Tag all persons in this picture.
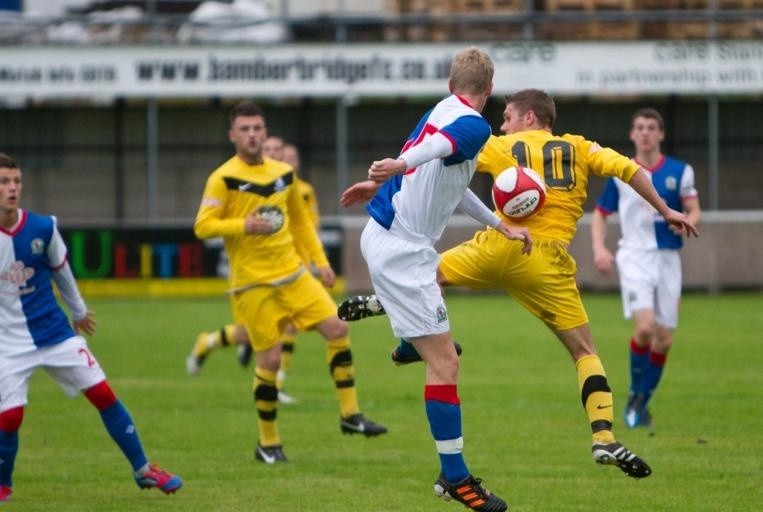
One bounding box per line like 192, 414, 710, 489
237, 145, 319, 366
195, 102, 388, 461
1, 152, 181, 499
187, 133, 333, 414
358, 47, 537, 510
590, 108, 702, 432
336, 87, 698, 480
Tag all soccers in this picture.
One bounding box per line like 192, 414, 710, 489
492, 165, 544, 220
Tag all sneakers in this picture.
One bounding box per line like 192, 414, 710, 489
134, 464, 183, 496
1, 486, 13, 501
624, 398, 643, 428
434, 473, 507, 512
340, 412, 388, 438
628, 395, 652, 426
254, 439, 287, 464
390, 342, 462, 366
592, 441, 652, 478
337, 294, 385, 322
186, 331, 297, 405
